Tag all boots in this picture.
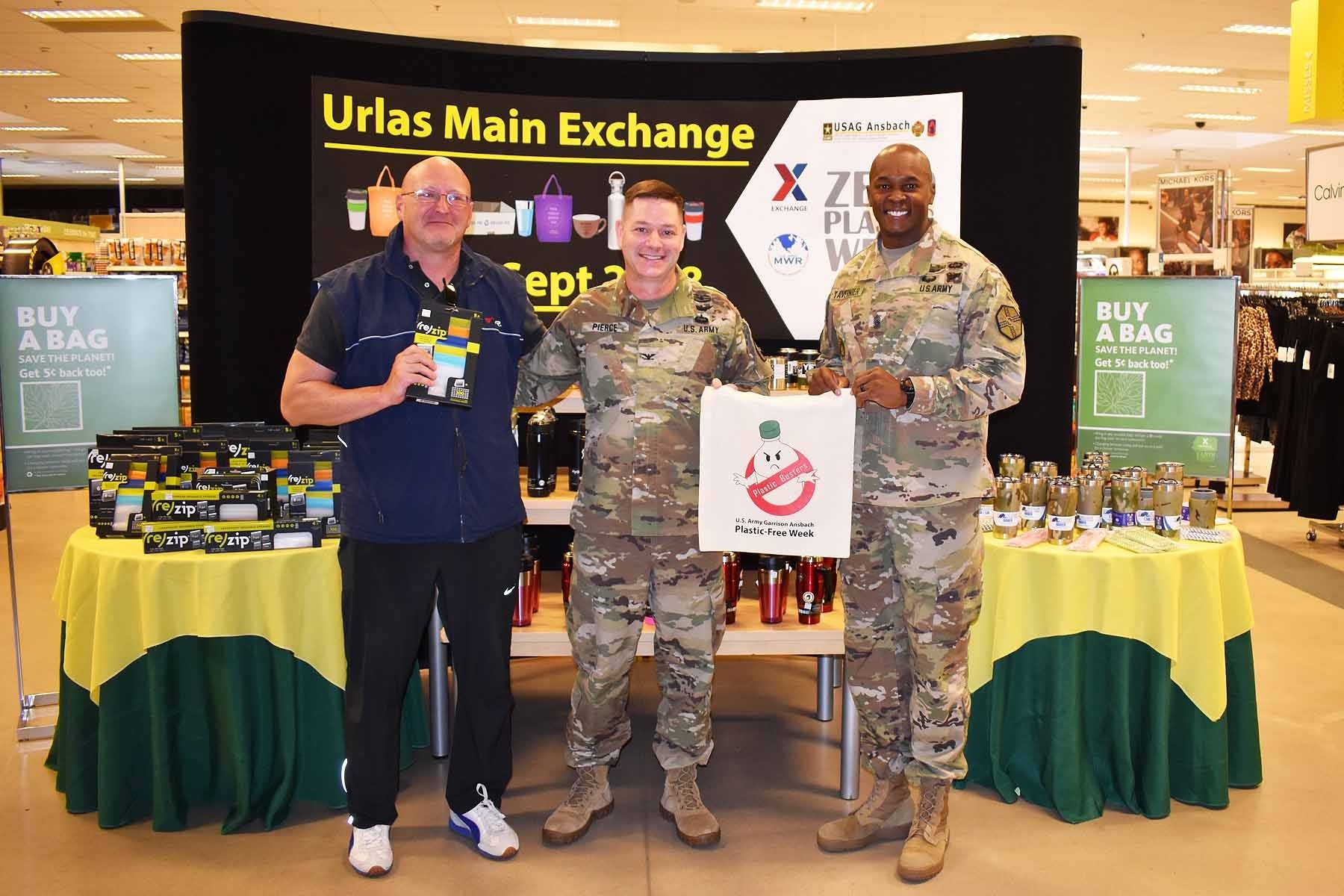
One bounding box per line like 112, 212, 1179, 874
899, 776, 951, 881
543, 765, 617, 844
659, 764, 721, 846
817, 768, 915, 852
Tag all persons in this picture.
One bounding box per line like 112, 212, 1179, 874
1127, 249, 1148, 276
1078, 217, 1090, 240
514, 180, 774, 848
809, 143, 1026, 884
1180, 188, 1213, 247
1089, 219, 1117, 239
280, 156, 547, 878
1265, 250, 1292, 269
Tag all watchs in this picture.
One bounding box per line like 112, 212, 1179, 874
900, 377, 915, 408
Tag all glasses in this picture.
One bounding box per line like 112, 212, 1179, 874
442, 277, 459, 306
401, 189, 475, 209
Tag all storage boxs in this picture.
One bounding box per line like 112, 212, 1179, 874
406, 300, 483, 410
87, 423, 341, 554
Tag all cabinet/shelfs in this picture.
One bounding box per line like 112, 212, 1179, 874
107, 262, 191, 404
428, 466, 862, 801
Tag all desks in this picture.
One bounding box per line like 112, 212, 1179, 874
951, 516, 1263, 822
43, 523, 428, 833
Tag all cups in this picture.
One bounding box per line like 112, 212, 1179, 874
573, 214, 606, 239
980, 451, 1217, 547
516, 200, 534, 237
510, 531, 575, 627
764, 355, 787, 391
347, 189, 367, 231
684, 201, 705, 241
722, 552, 836, 624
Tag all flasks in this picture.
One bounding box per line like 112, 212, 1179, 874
607, 171, 627, 250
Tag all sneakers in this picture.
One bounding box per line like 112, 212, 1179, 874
449, 785, 520, 862
348, 825, 393, 876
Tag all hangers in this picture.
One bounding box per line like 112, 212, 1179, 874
1239, 283, 1344, 327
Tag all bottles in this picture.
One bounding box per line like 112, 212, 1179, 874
510, 407, 587, 497
779, 347, 820, 392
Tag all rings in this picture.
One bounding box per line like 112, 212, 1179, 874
860, 385, 865, 392
